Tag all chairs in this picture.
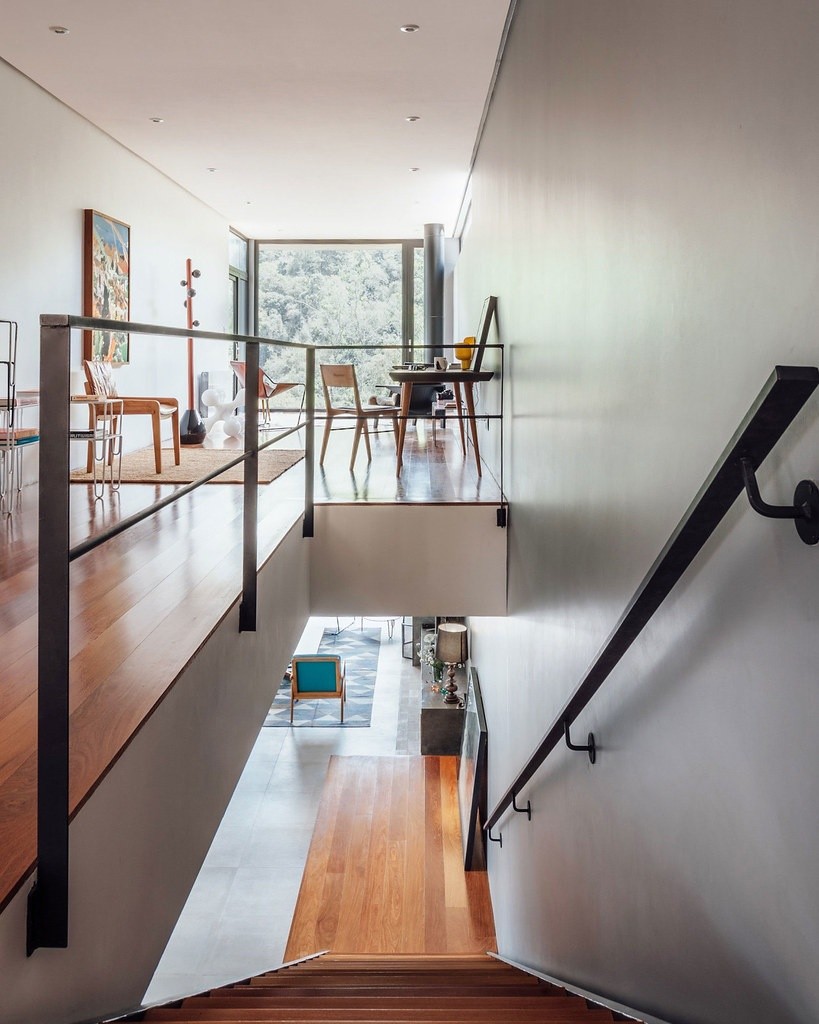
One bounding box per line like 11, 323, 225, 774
228, 358, 306, 433
319, 361, 401, 468
290, 653, 347, 725
82, 358, 181, 475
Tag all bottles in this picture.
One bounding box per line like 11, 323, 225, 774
433, 656, 444, 683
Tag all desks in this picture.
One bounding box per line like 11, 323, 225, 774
360, 617, 400, 639
388, 368, 495, 479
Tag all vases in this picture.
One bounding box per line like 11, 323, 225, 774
431, 665, 444, 682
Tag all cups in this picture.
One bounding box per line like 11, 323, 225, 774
434, 357, 446, 372
431, 682, 439, 694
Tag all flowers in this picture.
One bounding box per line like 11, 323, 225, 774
416, 634, 445, 671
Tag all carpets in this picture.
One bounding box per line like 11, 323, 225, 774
70, 447, 306, 484
262, 627, 382, 728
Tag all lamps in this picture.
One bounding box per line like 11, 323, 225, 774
434, 623, 469, 705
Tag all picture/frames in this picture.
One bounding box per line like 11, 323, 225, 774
85, 208, 132, 365
456, 666, 488, 871
468, 295, 497, 372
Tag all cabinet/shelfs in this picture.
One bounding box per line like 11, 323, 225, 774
0, 320, 124, 517
420, 622, 468, 756
